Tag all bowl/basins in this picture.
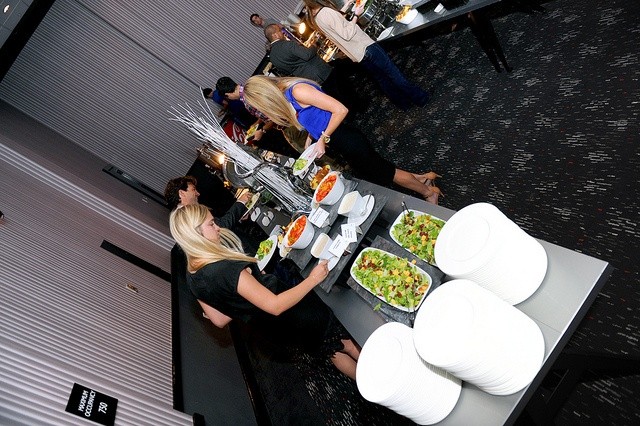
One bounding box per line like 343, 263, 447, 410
313, 170, 345, 205
284, 214, 315, 250
310, 233, 334, 261
396, 8, 418, 24
337, 191, 365, 218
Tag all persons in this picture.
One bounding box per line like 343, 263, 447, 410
243, 74, 445, 206
304, 0, 435, 110
203, 87, 259, 127
250, 14, 285, 30
264, 24, 367, 111
216, 77, 301, 159
169, 205, 360, 383
164, 176, 270, 258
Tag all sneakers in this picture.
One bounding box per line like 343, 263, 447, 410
420, 87, 434, 110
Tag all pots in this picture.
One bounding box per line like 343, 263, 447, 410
356, 0, 379, 26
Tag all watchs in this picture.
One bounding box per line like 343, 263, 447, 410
320, 131, 330, 144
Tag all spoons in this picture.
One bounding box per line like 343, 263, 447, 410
360, 192, 372, 216
262, 211, 274, 225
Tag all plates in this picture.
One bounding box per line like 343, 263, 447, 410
279, 233, 292, 257
316, 257, 340, 272
374, 26, 393, 41
244, 124, 259, 144
414, 280, 545, 397
250, 207, 261, 222
262, 207, 281, 226
260, 150, 267, 158
308, 166, 322, 181
434, 203, 547, 306
356, 322, 463, 426
292, 143, 319, 175
347, 195, 375, 227
434, 3, 444, 12
350, 247, 433, 313
323, 46, 338, 62
269, 225, 282, 239
284, 158, 295, 168
277, 231, 287, 248
298, 168, 309, 179
352, 0, 367, 12
237, 189, 249, 200
243, 192, 260, 217
254, 235, 277, 272
389, 208, 448, 268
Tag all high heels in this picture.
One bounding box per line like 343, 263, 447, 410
425, 186, 445, 205
423, 172, 442, 187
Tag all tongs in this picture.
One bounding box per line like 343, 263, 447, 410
401, 201, 416, 228
403, 281, 417, 327
